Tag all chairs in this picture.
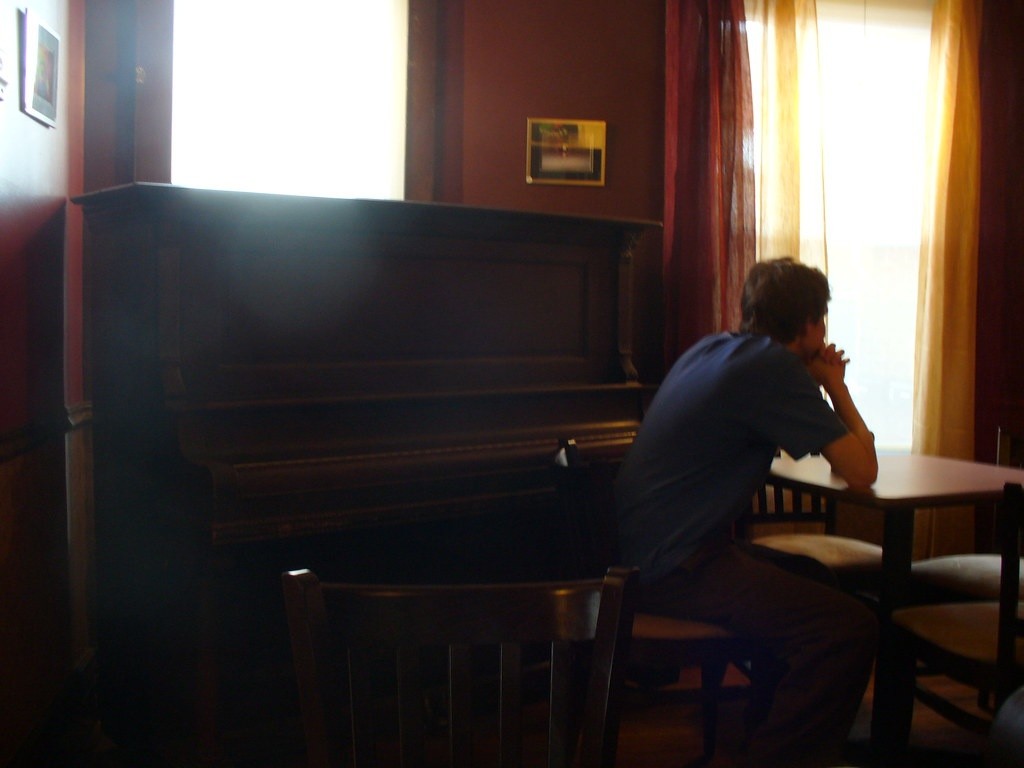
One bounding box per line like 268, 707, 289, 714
747, 447, 881, 689
548, 440, 742, 768
283, 565, 641, 768
881, 482, 1024, 768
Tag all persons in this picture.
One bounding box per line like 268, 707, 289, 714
613, 258, 880, 767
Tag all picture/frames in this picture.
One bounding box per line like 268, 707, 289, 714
524, 116, 609, 186
23, 7, 62, 130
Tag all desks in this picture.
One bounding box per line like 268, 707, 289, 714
764, 452, 1024, 768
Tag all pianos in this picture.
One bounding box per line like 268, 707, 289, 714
68, 182, 660, 768
